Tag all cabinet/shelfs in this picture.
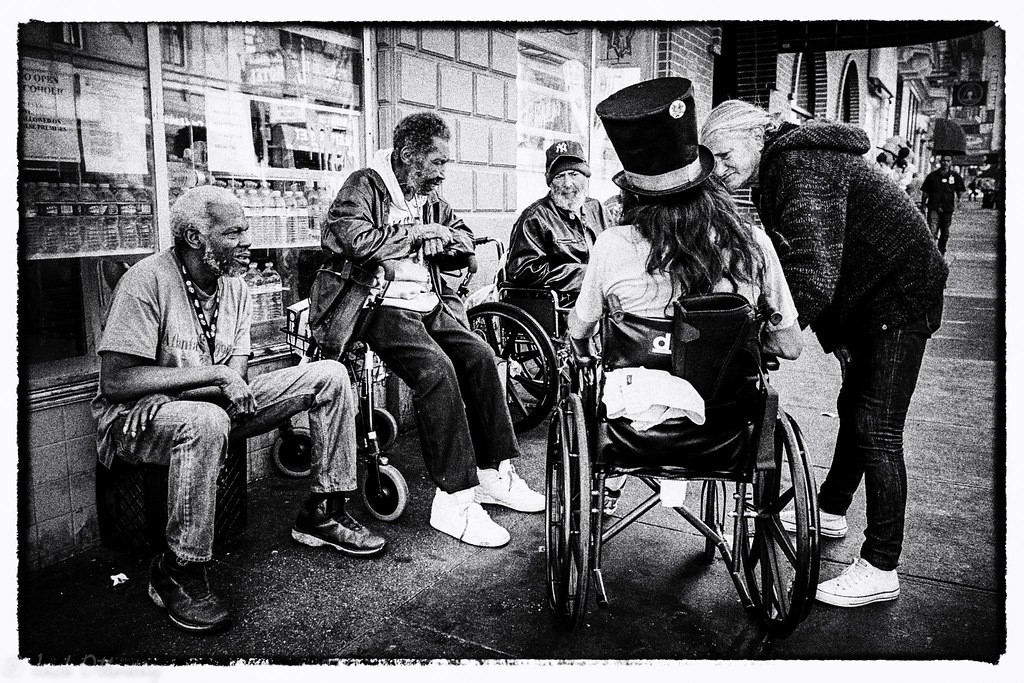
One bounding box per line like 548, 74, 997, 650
17, 21, 373, 259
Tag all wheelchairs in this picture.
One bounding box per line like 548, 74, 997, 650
270, 237, 572, 525
543, 289, 821, 635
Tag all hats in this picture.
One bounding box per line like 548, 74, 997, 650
897, 159, 908, 169
877, 153, 890, 164
595, 77, 717, 199
898, 147, 910, 158
545, 140, 592, 185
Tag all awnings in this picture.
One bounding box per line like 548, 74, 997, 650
934, 117, 966, 155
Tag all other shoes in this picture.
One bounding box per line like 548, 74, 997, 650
591, 472, 628, 516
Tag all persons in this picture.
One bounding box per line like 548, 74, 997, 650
702, 98, 949, 608
507, 141, 616, 312
875, 146, 979, 258
308, 112, 547, 548
564, 76, 805, 360
89, 185, 389, 633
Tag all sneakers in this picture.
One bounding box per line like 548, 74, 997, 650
815, 557, 900, 607
291, 492, 387, 554
148, 552, 229, 629
779, 507, 848, 538
474, 458, 545, 512
429, 487, 511, 547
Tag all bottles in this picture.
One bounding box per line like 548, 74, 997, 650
240, 262, 283, 322
20, 181, 155, 255
168, 137, 331, 243
